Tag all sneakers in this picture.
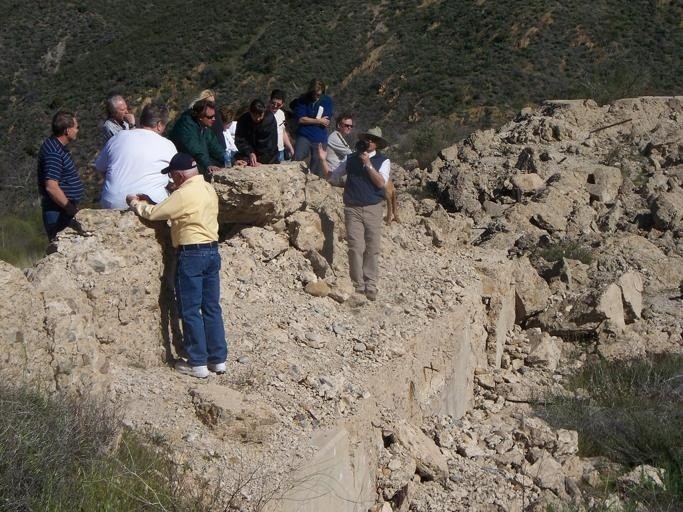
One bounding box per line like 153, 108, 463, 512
174, 359, 209, 379
367, 292, 378, 302
208, 361, 227, 375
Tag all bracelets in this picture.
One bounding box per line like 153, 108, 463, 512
367, 165, 373, 170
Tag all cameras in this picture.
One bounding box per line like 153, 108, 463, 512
356, 140, 370, 151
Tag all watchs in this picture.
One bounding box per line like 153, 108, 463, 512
129, 123, 136, 128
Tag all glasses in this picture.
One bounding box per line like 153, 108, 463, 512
202, 114, 218, 118
271, 100, 282, 107
340, 123, 355, 127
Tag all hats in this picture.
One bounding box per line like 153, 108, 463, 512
160, 152, 198, 174
358, 124, 390, 150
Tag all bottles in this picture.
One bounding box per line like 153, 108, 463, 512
223, 150, 231, 169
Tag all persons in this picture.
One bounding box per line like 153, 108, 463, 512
96, 103, 178, 209
103, 94, 137, 144
37, 110, 84, 242
164, 89, 297, 166
127, 152, 228, 378
328, 124, 390, 301
325, 113, 355, 187
292, 78, 333, 175
